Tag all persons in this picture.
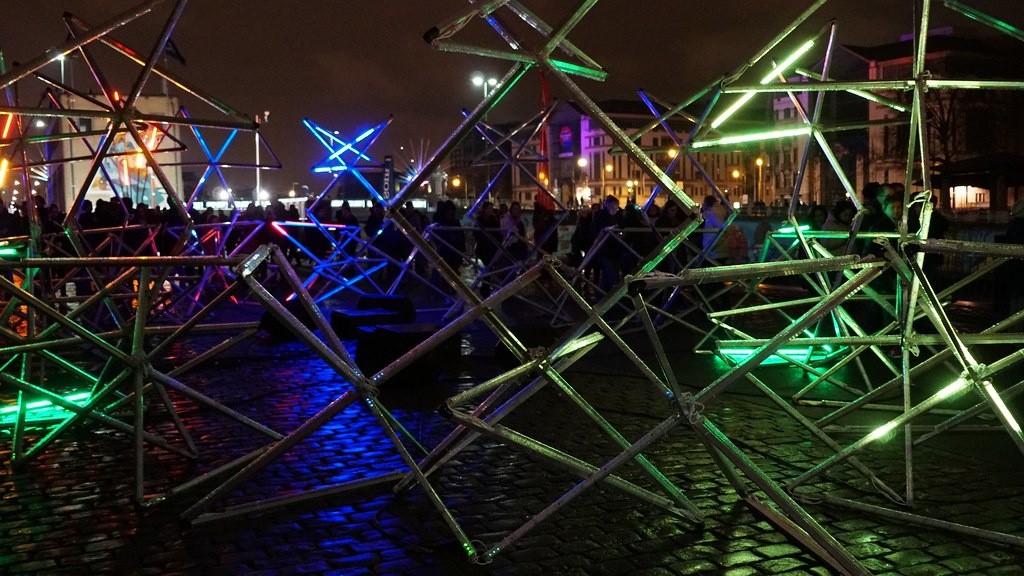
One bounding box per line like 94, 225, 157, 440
1, 180, 959, 333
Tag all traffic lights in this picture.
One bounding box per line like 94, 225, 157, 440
452, 177, 468, 200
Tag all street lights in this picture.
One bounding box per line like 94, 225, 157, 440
601, 163, 614, 202
469, 73, 498, 203
756, 158, 763, 202
